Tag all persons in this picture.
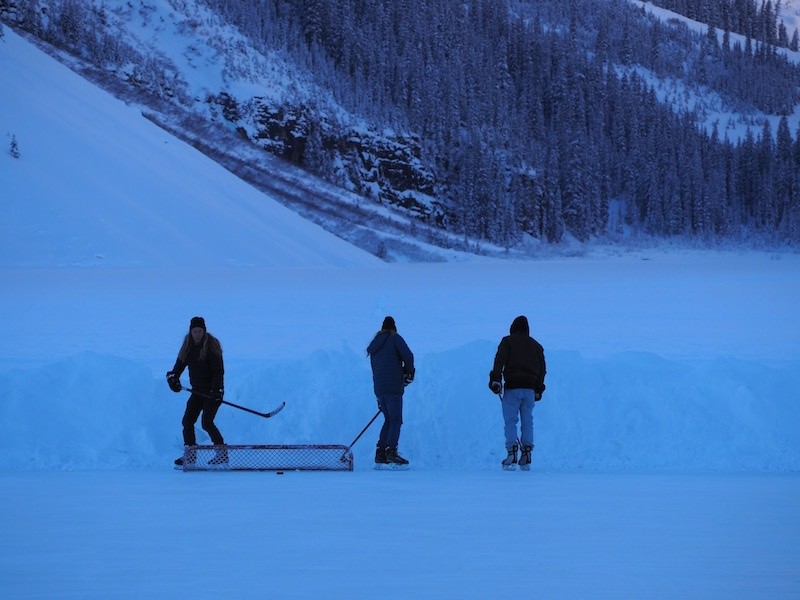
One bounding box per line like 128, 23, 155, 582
488, 316, 546, 471
166, 317, 228, 469
366, 316, 416, 471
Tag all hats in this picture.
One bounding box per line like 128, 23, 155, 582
190, 316, 206, 331
382, 316, 397, 332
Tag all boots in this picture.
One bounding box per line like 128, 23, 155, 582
518, 445, 531, 470
208, 443, 229, 464
376, 445, 387, 463
175, 443, 198, 465
385, 446, 410, 463
501, 446, 519, 470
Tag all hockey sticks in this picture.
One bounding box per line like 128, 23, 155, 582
499, 393, 526, 453
340, 409, 383, 463
169, 385, 286, 419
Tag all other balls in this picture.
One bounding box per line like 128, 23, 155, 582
277, 471, 283, 474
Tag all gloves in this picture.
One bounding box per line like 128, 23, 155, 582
166, 371, 181, 391
210, 388, 223, 401
491, 379, 501, 394
378, 399, 384, 412
402, 372, 415, 386
535, 384, 545, 401
